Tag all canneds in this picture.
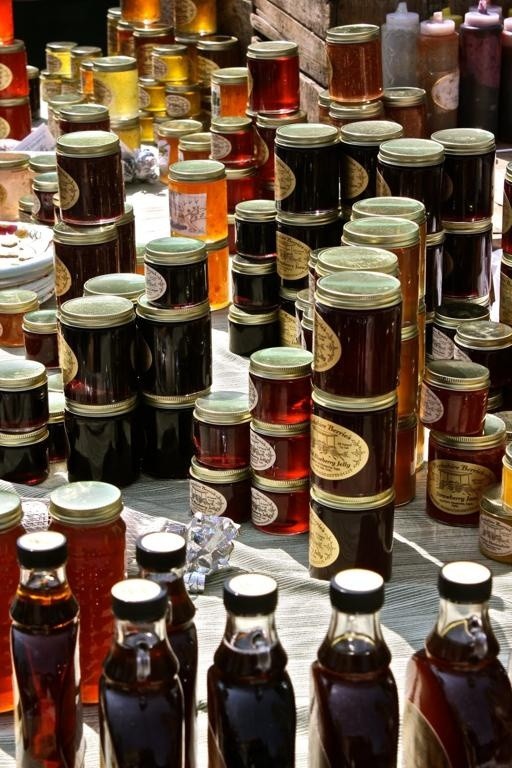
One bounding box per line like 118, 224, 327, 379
0, 488, 28, 713
0, 0, 512, 582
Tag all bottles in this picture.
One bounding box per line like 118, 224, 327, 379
12, 533, 84, 768
379, 0, 512, 145
306, 569, 402, 766
205, 575, 298, 767
402, 555, 512, 768
96, 577, 183, 766
134, 529, 196, 767
